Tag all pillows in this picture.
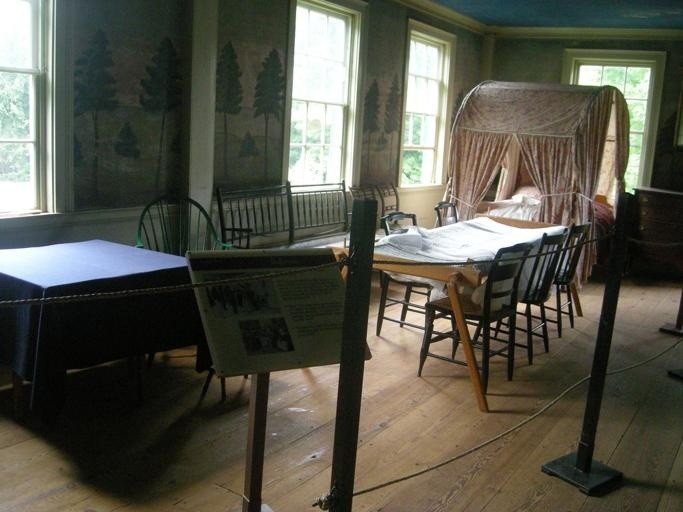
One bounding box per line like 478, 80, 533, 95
513, 186, 606, 219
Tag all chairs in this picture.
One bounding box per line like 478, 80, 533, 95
137, 195, 226, 401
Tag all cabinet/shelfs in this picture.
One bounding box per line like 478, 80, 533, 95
632, 187, 683, 277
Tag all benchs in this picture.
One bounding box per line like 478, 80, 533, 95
216, 180, 348, 250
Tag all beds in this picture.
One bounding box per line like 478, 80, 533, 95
434, 80, 630, 297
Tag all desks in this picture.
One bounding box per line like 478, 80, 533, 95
327, 216, 583, 413
0, 239, 214, 426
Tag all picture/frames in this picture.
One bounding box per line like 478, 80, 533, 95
673, 89, 683, 151
